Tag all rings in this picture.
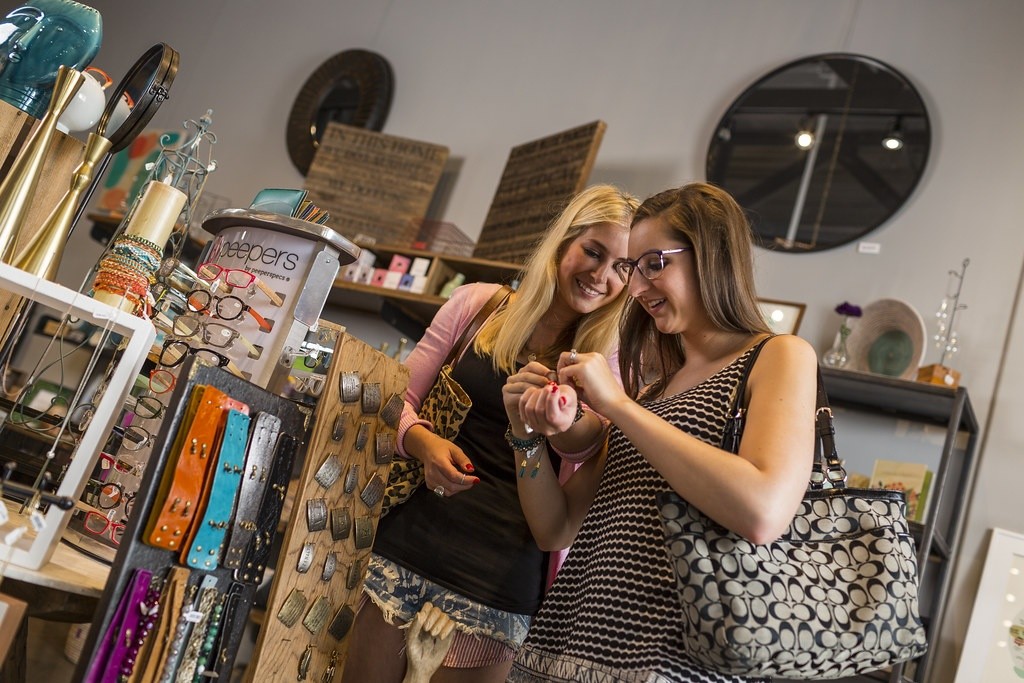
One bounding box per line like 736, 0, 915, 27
570, 348, 577, 364
460, 474, 466, 484
433, 485, 445, 498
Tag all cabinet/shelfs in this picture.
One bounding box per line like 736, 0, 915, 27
44, 215, 983, 683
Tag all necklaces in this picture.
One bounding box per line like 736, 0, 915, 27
522, 333, 549, 363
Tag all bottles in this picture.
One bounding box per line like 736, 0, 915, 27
438, 272, 465, 300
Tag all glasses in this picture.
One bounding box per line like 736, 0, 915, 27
611, 247, 690, 285
85, 454, 148, 547
118, 395, 171, 451
147, 262, 283, 393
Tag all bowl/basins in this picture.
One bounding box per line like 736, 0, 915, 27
830, 297, 928, 380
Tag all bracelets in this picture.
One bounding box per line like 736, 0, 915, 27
568, 400, 585, 431
504, 421, 547, 479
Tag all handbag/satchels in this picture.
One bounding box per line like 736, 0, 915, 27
655, 333, 930, 680
379, 283, 517, 521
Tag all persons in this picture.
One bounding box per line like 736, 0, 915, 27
502, 181, 819, 683
342, 185, 642, 683
400, 601, 456, 683
